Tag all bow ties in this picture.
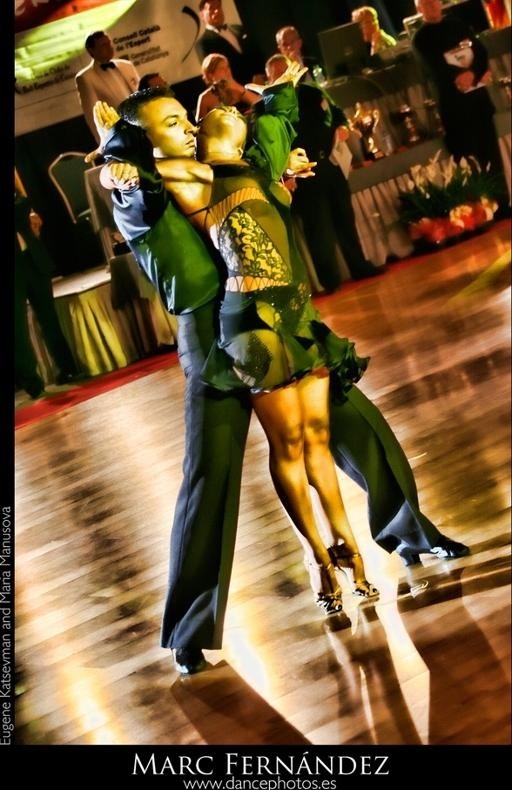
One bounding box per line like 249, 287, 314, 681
100, 62, 116, 71
215, 23, 227, 32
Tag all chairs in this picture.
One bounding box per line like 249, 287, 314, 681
47, 149, 114, 250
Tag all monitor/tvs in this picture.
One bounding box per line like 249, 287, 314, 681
317, 21, 365, 81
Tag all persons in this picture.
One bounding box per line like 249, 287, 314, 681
84, 84, 471, 675
99, 59, 381, 619
14, 0, 512, 403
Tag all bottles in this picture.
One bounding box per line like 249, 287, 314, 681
311, 63, 328, 86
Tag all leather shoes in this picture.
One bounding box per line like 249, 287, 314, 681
172, 646, 206, 674
395, 533, 470, 566
54, 369, 79, 386
323, 282, 340, 291
30, 384, 45, 399
352, 260, 387, 281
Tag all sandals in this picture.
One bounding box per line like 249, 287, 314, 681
303, 553, 342, 615
327, 543, 379, 597
